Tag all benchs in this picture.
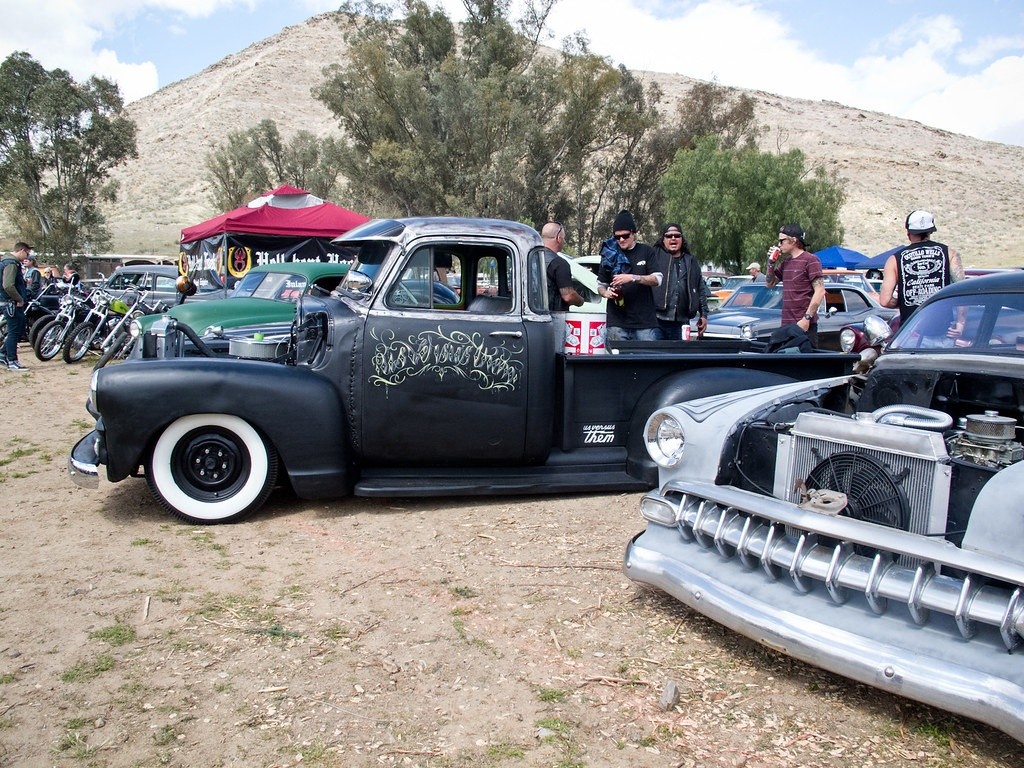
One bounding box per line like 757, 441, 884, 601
467, 293, 511, 312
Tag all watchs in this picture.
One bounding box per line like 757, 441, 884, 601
804, 314, 812, 320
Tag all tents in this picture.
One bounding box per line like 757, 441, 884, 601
812, 244, 908, 271
177, 183, 452, 307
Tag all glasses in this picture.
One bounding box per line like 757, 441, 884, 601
780, 238, 787, 244
21, 249, 30, 257
554, 221, 563, 240
664, 234, 682, 238
614, 230, 632, 240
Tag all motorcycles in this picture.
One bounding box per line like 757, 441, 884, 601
25, 272, 173, 374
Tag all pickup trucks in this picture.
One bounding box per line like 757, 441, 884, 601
72, 217, 868, 526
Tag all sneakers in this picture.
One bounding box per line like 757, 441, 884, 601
7, 360, 30, 372
0, 353, 8, 365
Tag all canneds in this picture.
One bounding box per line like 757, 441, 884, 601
769, 247, 781, 261
682, 325, 691, 341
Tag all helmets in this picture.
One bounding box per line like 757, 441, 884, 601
175, 276, 197, 296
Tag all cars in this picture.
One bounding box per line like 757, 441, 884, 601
623, 269, 1024, 738
103, 237, 320, 316
690, 279, 907, 351
131, 259, 362, 360
358, 262, 465, 307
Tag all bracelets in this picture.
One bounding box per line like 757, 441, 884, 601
632, 274, 636, 283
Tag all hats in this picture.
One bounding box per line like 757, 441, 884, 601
906, 210, 937, 235
663, 223, 682, 235
779, 223, 811, 247
745, 263, 761, 271
613, 210, 636, 235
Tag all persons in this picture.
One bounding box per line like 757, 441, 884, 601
0, 241, 33, 370
746, 263, 766, 283
541, 222, 583, 352
596, 210, 664, 341
649, 223, 709, 340
766, 224, 826, 350
22, 254, 80, 299
880, 209, 968, 349
